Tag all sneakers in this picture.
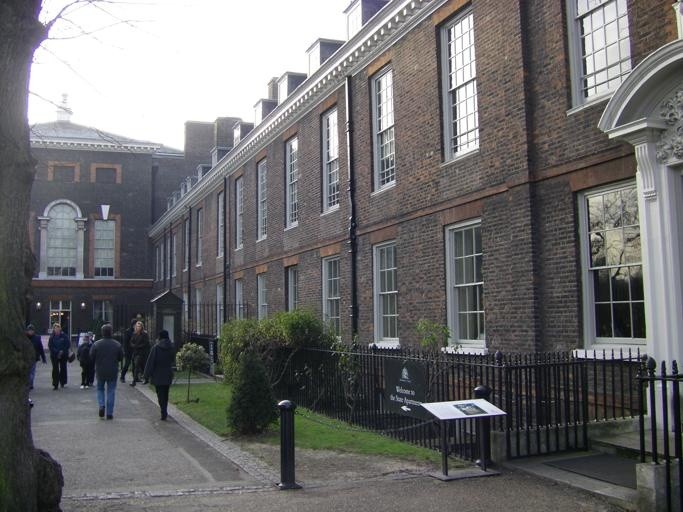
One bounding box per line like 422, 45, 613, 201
53, 384, 64, 390
99, 407, 113, 419
160, 409, 166, 419
80, 385, 89, 390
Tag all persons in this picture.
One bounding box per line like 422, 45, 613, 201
87, 330, 96, 384
128, 321, 150, 387
26, 325, 48, 388
75, 336, 92, 388
119, 317, 139, 384
89, 324, 124, 420
143, 330, 177, 419
48, 322, 72, 390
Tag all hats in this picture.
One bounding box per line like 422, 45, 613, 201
27, 324, 36, 330
159, 330, 168, 339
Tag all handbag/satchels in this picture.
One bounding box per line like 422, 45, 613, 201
68, 351, 75, 362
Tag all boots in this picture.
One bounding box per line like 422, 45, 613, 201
121, 369, 148, 386
